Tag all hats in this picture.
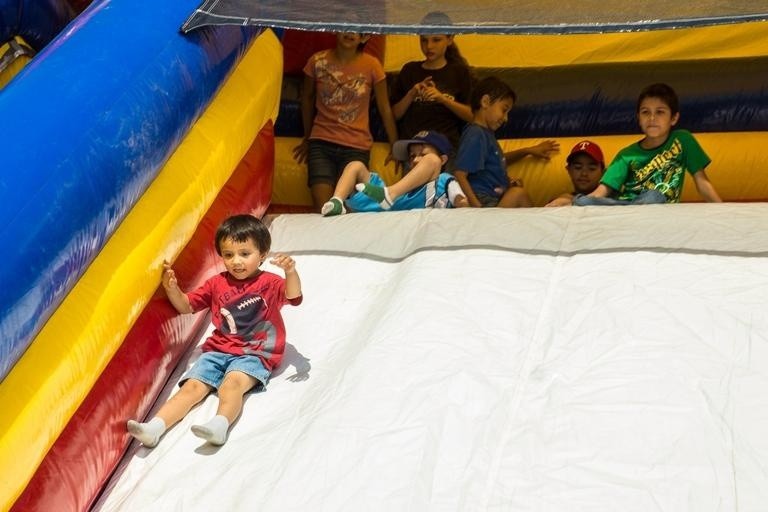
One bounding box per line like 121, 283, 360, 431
393, 130, 449, 160
566, 141, 604, 164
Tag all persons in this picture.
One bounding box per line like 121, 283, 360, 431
494, 140, 606, 207
453, 75, 561, 207
125, 214, 303, 448
291, 2, 399, 213
569, 81, 723, 204
320, 129, 469, 213
381, 11, 472, 160
